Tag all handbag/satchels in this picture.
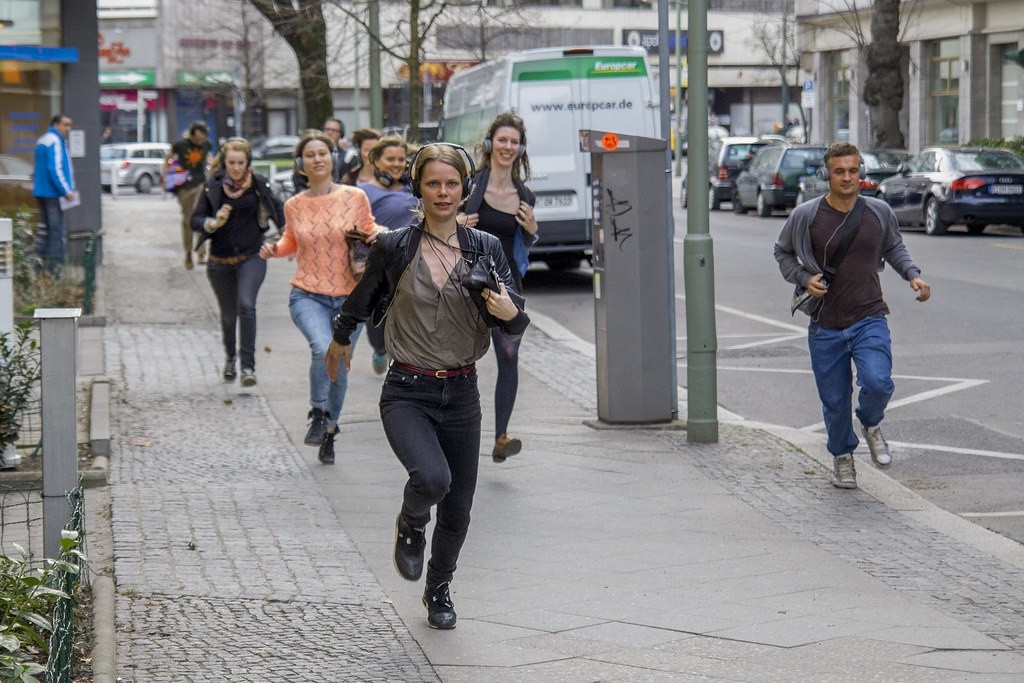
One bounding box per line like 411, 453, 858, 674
790, 266, 830, 322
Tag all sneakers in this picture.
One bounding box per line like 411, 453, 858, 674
393, 513, 432, 581
303, 406, 340, 464
860, 424, 892, 469
832, 451, 856, 489
492, 434, 522, 463
421, 581, 458, 630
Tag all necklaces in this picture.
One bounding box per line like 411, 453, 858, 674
310, 183, 334, 196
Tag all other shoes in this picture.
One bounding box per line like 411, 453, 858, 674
184, 252, 208, 270
224, 355, 236, 381
372, 350, 387, 377
239, 367, 257, 388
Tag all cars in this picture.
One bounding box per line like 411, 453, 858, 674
267, 169, 297, 200
248, 135, 302, 160
729, 146, 828, 217
680, 137, 790, 210
1, 156, 43, 222
764, 123, 807, 145
874, 145, 1024, 236
100, 142, 172, 195
794, 151, 914, 206
376, 121, 439, 144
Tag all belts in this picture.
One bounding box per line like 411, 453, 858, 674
394, 360, 475, 378
208, 254, 246, 265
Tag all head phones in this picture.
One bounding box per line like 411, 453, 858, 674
319, 116, 345, 139
482, 121, 527, 157
817, 145, 866, 181
408, 142, 475, 199
294, 144, 338, 170
372, 157, 410, 186
219, 137, 252, 171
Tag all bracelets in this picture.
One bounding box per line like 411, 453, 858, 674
205, 218, 217, 233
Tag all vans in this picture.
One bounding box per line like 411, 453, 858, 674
440, 43, 661, 273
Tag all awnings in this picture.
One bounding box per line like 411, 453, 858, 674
0, 45, 79, 62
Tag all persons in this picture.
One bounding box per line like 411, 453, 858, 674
774, 142, 931, 488
163, 120, 213, 271
33, 115, 75, 280
456, 113, 540, 463
325, 144, 530, 629
259, 133, 390, 466
292, 118, 424, 376
100, 124, 114, 193
190, 136, 285, 387
